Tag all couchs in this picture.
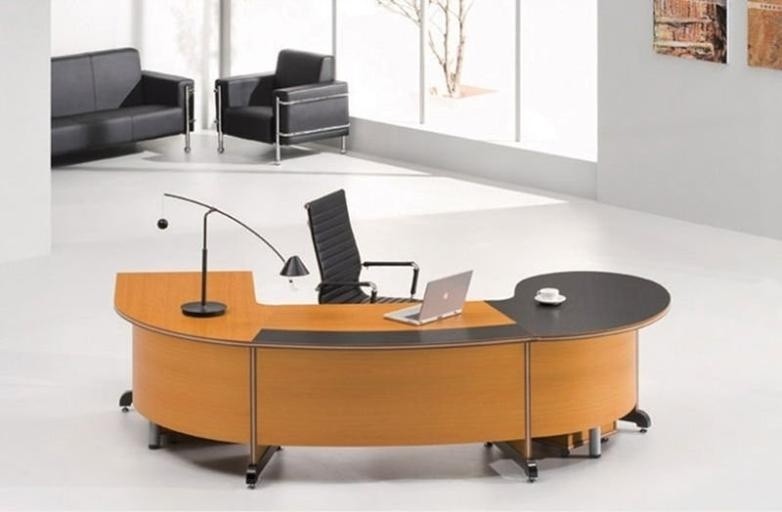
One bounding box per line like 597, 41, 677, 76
51, 48, 196, 163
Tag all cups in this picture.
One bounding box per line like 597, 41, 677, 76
537, 287, 559, 300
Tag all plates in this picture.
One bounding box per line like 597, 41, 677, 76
533, 295, 566, 306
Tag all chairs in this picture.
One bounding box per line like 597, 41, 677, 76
304, 188, 425, 306
214, 49, 349, 167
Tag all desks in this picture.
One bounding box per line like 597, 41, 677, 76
112, 272, 673, 489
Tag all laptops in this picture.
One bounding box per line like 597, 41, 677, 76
384, 270, 473, 326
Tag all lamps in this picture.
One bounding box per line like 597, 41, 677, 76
158, 190, 310, 315
